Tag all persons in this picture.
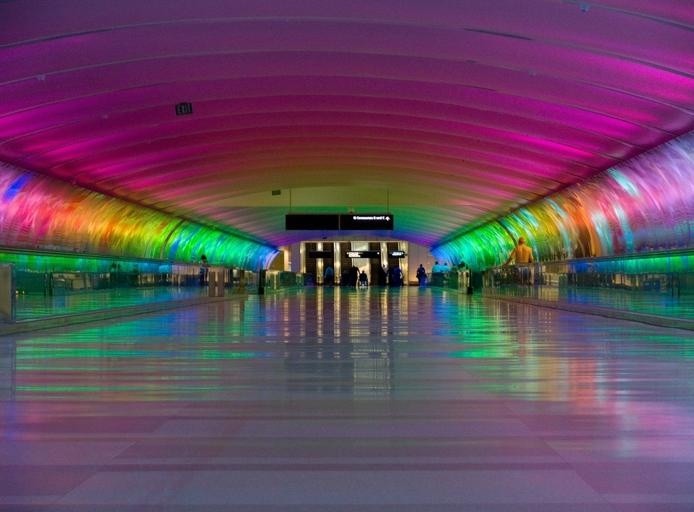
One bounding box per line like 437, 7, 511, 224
503, 237, 533, 266
319, 261, 457, 288
198, 255, 211, 285
